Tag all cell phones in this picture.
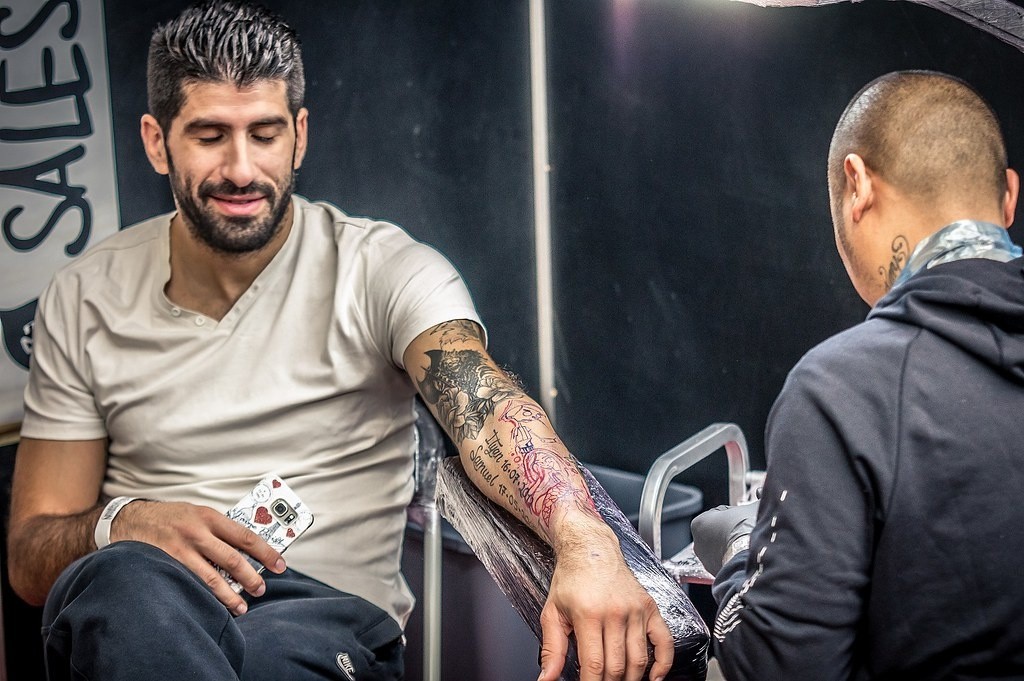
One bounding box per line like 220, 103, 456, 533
202, 473, 314, 601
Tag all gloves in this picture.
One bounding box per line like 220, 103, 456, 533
690, 486, 764, 578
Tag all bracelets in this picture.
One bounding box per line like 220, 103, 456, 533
93, 496, 149, 551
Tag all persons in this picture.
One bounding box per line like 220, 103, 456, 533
7, 2, 675, 680
690, 69, 1023, 681
